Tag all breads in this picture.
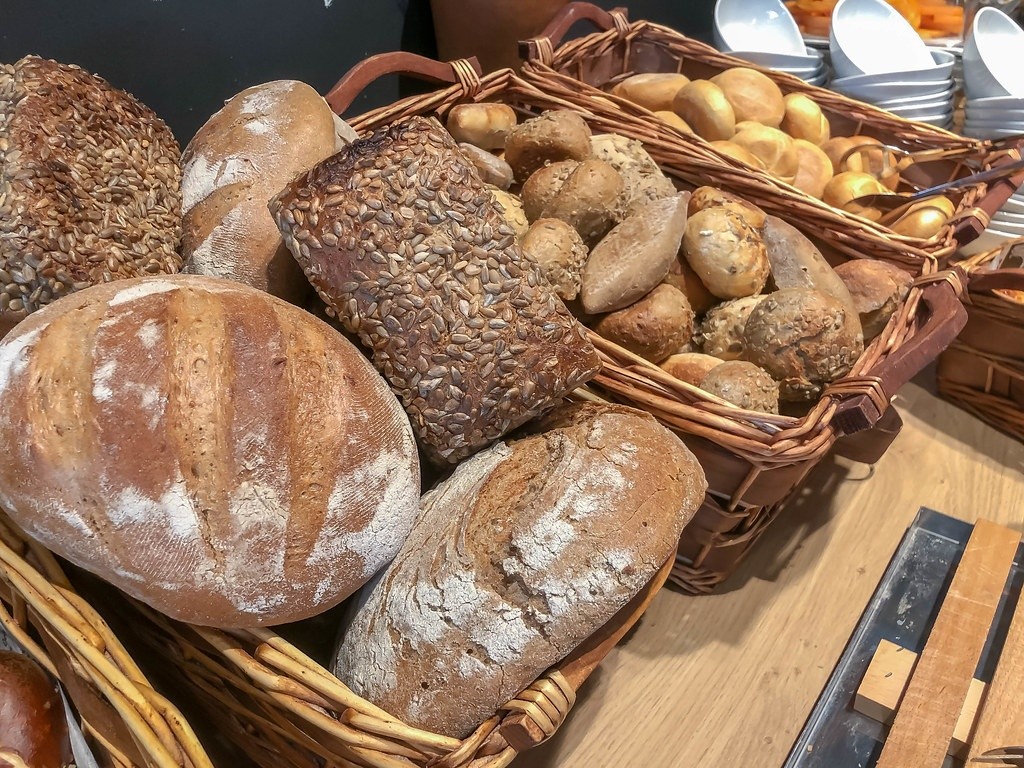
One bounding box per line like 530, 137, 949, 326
0, 53, 957, 767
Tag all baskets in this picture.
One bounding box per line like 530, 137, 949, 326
0, 509, 217, 768
116, 378, 678, 768
516, 0, 1024, 275
323, 51, 968, 595
938, 236, 1024, 445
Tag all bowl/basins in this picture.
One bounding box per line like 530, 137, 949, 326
709, 1, 1023, 258
